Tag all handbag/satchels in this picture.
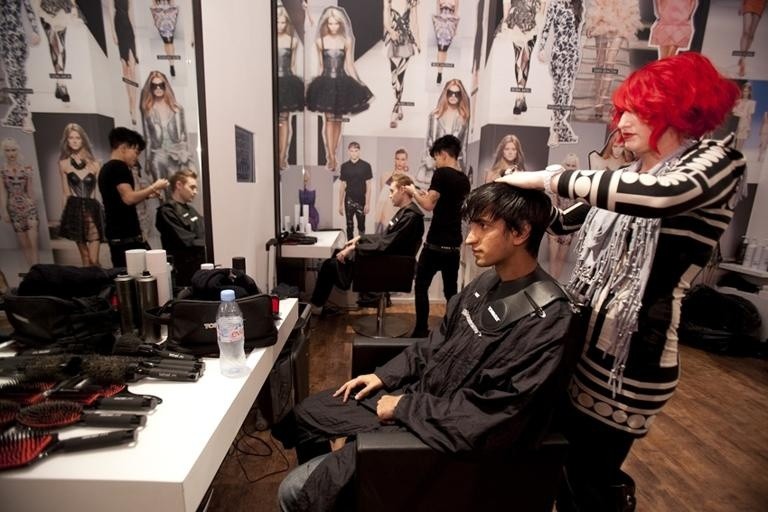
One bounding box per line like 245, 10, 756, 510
147, 269, 278, 356
4, 264, 125, 347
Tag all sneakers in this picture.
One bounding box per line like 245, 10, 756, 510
310, 304, 323, 315
348, 307, 379, 316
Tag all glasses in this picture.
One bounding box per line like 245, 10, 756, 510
447, 90, 462, 97
150, 82, 166, 90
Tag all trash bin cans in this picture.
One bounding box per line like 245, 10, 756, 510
255, 302, 311, 431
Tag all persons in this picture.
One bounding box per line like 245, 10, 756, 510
2, 1, 107, 267
107, 1, 179, 125
308, 174, 426, 316
402, 135, 471, 338
421, 78, 471, 219
380, 149, 416, 229
129, 159, 156, 242
486, 135, 527, 184
140, 70, 196, 207
276, 2, 768, 171
98, 127, 171, 269
299, 170, 320, 231
156, 168, 207, 268
274, 182, 584, 512
494, 50, 748, 512
339, 141, 373, 245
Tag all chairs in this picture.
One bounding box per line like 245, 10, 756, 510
351, 304, 593, 510
351, 232, 423, 339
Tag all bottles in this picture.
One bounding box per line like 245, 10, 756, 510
215, 289, 247, 378
113, 268, 160, 343
284, 215, 291, 232
737, 234, 767, 271
298, 217, 313, 232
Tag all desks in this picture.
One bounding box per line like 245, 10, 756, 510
281, 231, 343, 259
1, 297, 301, 512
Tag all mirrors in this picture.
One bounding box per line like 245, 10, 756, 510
270, 0, 309, 238
0, 0, 216, 337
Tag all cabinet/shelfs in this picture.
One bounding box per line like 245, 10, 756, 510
717, 261, 767, 342
